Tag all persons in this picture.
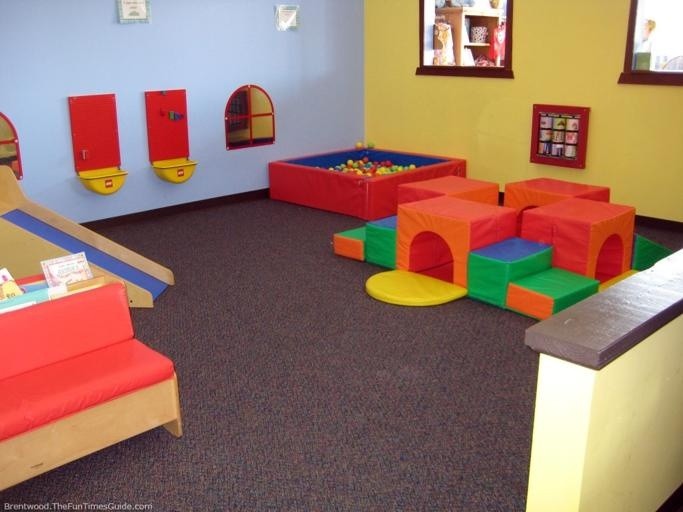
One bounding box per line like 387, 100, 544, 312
267, 140, 674, 321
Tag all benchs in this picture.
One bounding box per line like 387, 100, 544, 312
0, 276, 183, 490
14, 274, 49, 292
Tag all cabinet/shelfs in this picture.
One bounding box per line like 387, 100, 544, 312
436, 7, 501, 67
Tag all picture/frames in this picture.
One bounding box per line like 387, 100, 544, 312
116, 0, 151, 24
531, 104, 589, 168
276, 6, 300, 29
